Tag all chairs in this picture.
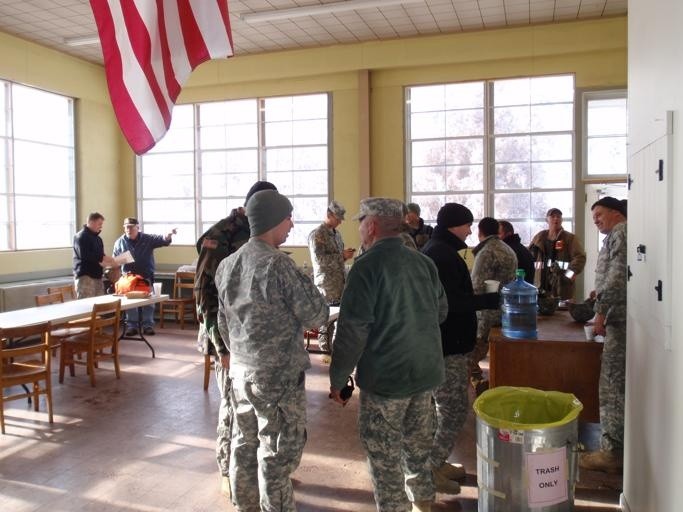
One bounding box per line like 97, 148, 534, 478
159, 271, 195, 331
0, 284, 123, 435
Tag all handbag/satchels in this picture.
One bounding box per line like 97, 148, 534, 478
115, 273, 151, 295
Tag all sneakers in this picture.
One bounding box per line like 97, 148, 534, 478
144, 327, 154, 335
126, 329, 137, 336
433, 471, 460, 495
222, 476, 232, 499
440, 461, 466, 479
578, 449, 624, 473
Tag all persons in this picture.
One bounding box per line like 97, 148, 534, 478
109, 216, 177, 337
328, 195, 448, 511
397, 200, 535, 495
528, 208, 585, 303
576, 197, 627, 472
72, 214, 121, 301
192, 180, 278, 498
308, 203, 353, 365
211, 188, 331, 512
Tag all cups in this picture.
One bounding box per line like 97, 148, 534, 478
484, 280, 500, 293
152, 283, 162, 296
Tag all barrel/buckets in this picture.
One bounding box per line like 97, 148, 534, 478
500, 269, 539, 339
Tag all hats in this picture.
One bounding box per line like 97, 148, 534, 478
547, 208, 562, 216
123, 218, 140, 227
437, 204, 473, 228
537, 298, 560, 314
244, 181, 277, 207
353, 198, 404, 220
328, 201, 345, 220
568, 299, 596, 322
244, 189, 293, 237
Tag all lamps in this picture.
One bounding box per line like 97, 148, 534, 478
240, 0, 418, 26
62, 35, 99, 49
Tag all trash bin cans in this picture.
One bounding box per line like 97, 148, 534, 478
472, 387, 584, 512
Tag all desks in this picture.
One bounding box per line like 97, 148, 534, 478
0, 285, 169, 404
485, 309, 602, 425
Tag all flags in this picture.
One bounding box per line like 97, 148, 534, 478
88, 0, 241, 156
